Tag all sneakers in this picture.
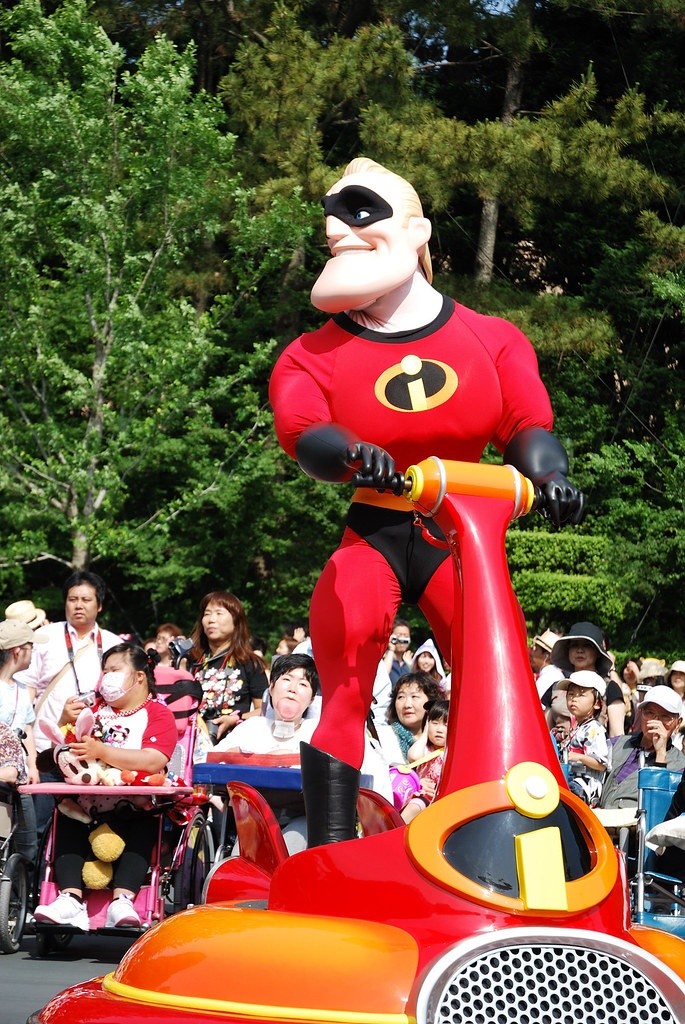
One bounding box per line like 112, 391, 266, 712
105, 893, 141, 928
34, 890, 90, 932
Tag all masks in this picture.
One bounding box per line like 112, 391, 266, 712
99, 669, 139, 702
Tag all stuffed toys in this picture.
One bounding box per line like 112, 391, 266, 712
34, 709, 165, 888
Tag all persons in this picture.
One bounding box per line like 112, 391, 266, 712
0, 572, 685, 936
266, 157, 588, 852
33, 641, 178, 928
212, 653, 324, 857
548, 670, 613, 810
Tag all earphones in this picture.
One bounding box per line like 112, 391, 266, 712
546, 654, 549, 658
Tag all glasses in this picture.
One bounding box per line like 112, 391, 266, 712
12, 644, 36, 652
641, 708, 678, 726
155, 635, 170, 643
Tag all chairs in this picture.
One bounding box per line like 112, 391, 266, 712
633, 750, 685, 940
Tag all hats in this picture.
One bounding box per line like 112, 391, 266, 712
636, 660, 666, 683
550, 621, 612, 678
0, 619, 49, 650
638, 685, 682, 717
668, 660, 685, 674
5, 600, 46, 629
556, 670, 605, 698
532, 629, 560, 654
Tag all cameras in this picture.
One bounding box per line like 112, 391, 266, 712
72, 691, 96, 707
168, 638, 194, 659
390, 637, 410, 645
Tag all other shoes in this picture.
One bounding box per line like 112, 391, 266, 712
651, 901, 670, 914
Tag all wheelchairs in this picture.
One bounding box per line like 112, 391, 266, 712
0, 781, 29, 954
32, 678, 209, 961
184, 753, 375, 906
